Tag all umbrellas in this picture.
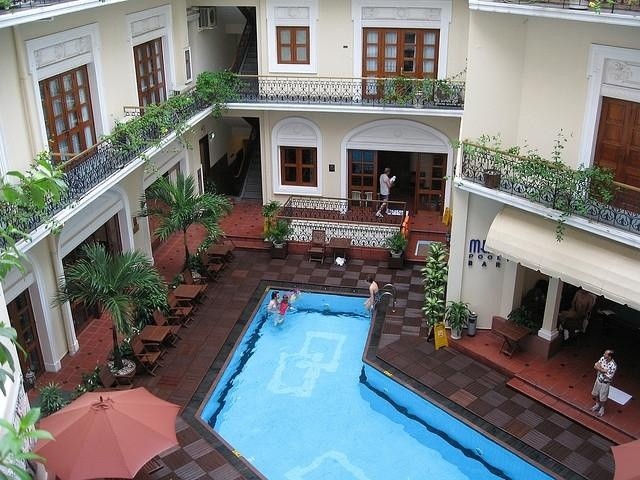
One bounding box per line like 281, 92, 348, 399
29, 386, 186, 480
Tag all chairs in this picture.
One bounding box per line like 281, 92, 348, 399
99, 237, 235, 391
519, 278, 547, 330
103, 453, 164, 480
308, 230, 327, 264
557, 290, 598, 353
350, 191, 380, 208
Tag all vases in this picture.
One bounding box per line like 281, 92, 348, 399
483, 169, 500, 188
413, 89, 425, 108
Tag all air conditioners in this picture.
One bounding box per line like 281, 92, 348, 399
175, 85, 188, 95
199, 6, 216, 28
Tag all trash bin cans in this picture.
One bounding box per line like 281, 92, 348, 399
467, 312, 477, 336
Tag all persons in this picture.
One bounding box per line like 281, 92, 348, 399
362, 275, 380, 311
591, 348, 617, 417
571, 289, 594, 334
274, 292, 291, 327
25, 366, 38, 390
267, 289, 281, 313
374, 167, 396, 219
289, 287, 302, 303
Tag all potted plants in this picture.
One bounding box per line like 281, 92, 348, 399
421, 297, 444, 336
444, 300, 471, 340
50, 242, 171, 385
386, 235, 408, 259
261, 200, 294, 249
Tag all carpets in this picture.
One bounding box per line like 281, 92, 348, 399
605, 385, 634, 407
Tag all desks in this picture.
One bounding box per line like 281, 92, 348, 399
327, 237, 352, 263
494, 322, 533, 359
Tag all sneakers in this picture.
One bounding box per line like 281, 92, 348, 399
591, 406, 600, 411
376, 213, 384, 218
597, 410, 604, 417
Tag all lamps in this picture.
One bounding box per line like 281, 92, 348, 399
123, 105, 145, 117
